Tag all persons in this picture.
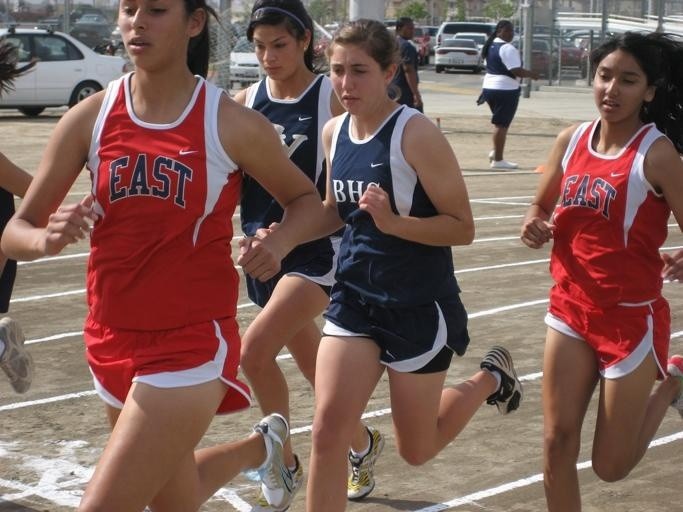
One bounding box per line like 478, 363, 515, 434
385, 16, 423, 115
476, 20, 540, 169
220, 0, 382, 508
251, 19, 523, 512
0, 36, 36, 398
0, 0, 322, 509
519, 29, 681, 511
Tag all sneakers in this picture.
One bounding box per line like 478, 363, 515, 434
251, 411, 294, 509
490, 159, 519, 170
661, 352, 683, 419
489, 149, 496, 163
346, 425, 385, 501
1, 317, 35, 393
249, 453, 304, 512
479, 345, 523, 417
